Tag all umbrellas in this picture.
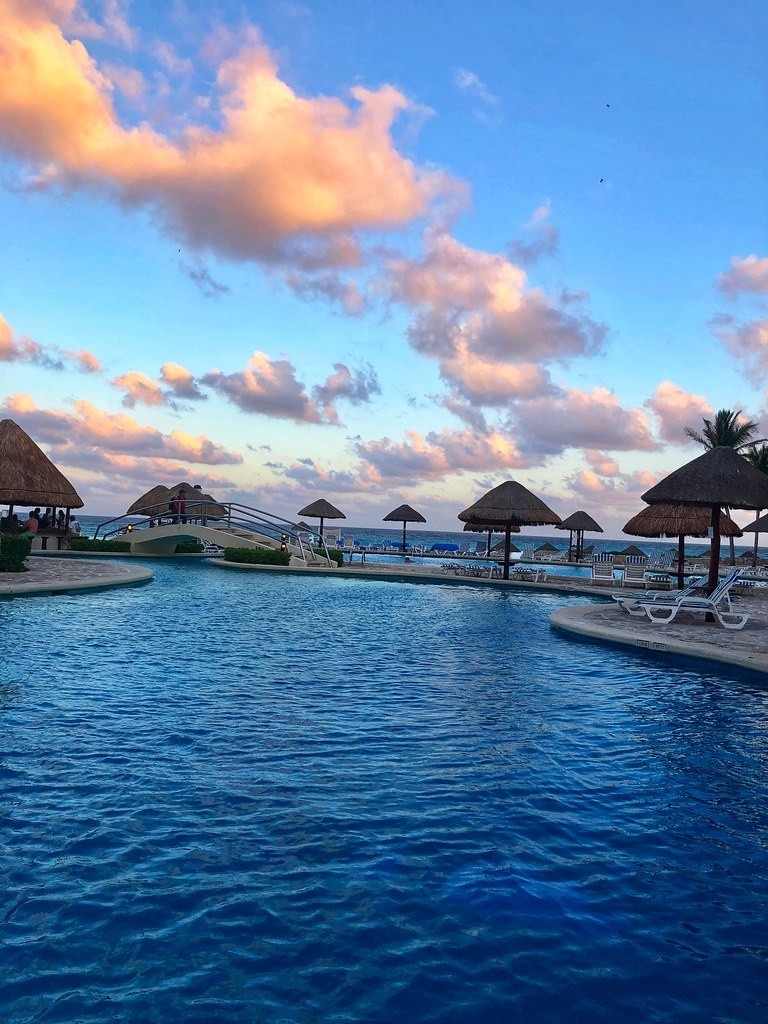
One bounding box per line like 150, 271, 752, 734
0, 419, 85, 533
739, 513, 768, 535
463, 523, 521, 557
382, 504, 427, 551
127, 482, 224, 525
554, 511, 605, 562
457, 481, 562, 581
291, 521, 312, 531
640, 447, 768, 625
297, 499, 346, 547
620, 505, 743, 590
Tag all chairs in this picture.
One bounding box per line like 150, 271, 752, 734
202, 538, 225, 554
298, 531, 752, 629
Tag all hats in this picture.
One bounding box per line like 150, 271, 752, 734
69, 515, 75, 519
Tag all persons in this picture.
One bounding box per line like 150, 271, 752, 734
2, 508, 81, 550
404, 557, 415, 563
170, 489, 188, 524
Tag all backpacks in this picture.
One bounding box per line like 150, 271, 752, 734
73, 521, 81, 532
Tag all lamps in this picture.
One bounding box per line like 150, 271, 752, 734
128, 524, 133, 532
280, 533, 286, 541
281, 542, 286, 552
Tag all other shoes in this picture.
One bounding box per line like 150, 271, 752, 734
66, 547, 71, 550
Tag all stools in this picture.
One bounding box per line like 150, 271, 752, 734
56, 537, 65, 550
41, 537, 50, 550
27, 536, 36, 543
